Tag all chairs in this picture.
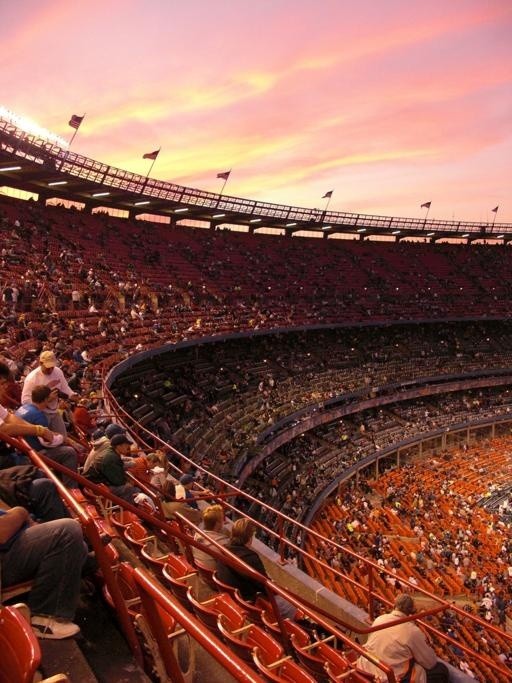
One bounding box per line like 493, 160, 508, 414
0, 194, 511, 683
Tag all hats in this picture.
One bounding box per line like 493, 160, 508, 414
181, 474, 197, 484
40, 350, 59, 369
105, 423, 133, 446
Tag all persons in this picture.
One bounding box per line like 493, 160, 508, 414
0, 194, 512, 682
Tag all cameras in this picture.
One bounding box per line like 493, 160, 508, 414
52, 387, 68, 400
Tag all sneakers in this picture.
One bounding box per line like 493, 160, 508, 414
31, 616, 80, 640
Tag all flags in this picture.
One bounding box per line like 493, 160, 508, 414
142, 150, 159, 161
321, 191, 332, 198
216, 171, 230, 180
69, 114, 84, 129
